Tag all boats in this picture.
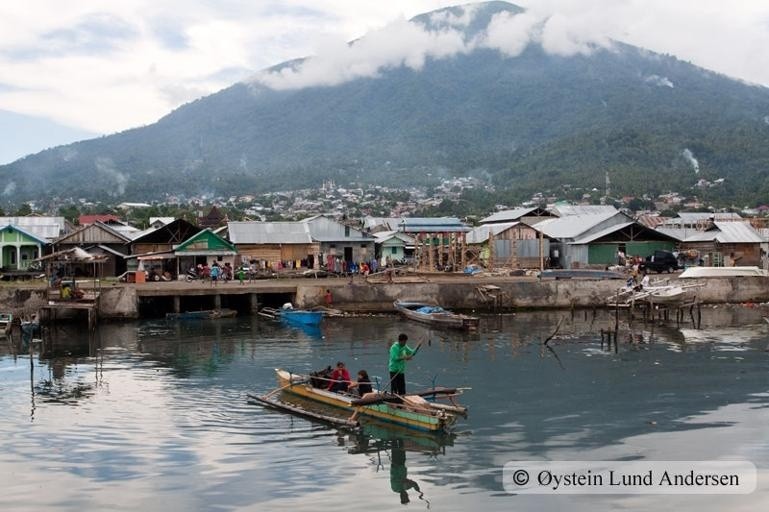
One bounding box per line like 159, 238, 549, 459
166, 308, 237, 319
280, 306, 323, 326
617, 284, 685, 303
274, 368, 452, 440
392, 297, 480, 330
0, 312, 39, 339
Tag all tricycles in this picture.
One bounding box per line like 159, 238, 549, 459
642, 251, 684, 275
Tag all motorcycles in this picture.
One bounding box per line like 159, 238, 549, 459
183, 265, 206, 282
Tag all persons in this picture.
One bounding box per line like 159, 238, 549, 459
699, 251, 714, 269
553, 248, 560, 264
144, 264, 172, 282
359, 262, 370, 280
331, 361, 350, 389
61, 284, 73, 300
723, 253, 737, 266
190, 261, 232, 287
250, 259, 261, 280
238, 262, 245, 285
388, 333, 422, 405
350, 370, 372, 398
31, 315, 38, 324
618, 252, 649, 291
325, 289, 332, 309
